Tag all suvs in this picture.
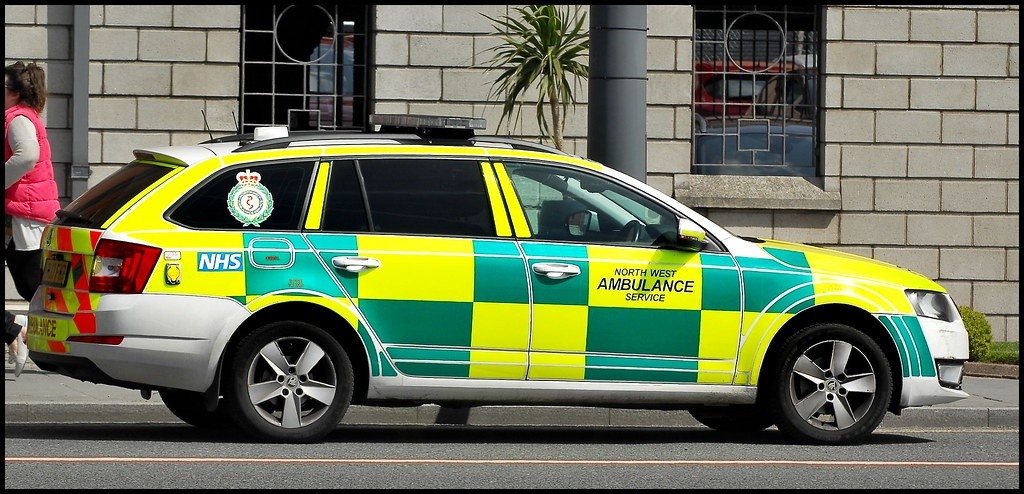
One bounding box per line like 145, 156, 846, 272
27, 114, 972, 445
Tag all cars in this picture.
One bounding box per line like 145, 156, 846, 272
308, 37, 355, 124
695, 65, 817, 179
696, 62, 797, 113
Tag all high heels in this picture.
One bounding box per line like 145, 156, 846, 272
8, 322, 29, 378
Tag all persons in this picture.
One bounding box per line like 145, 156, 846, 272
5, 60, 61, 377
